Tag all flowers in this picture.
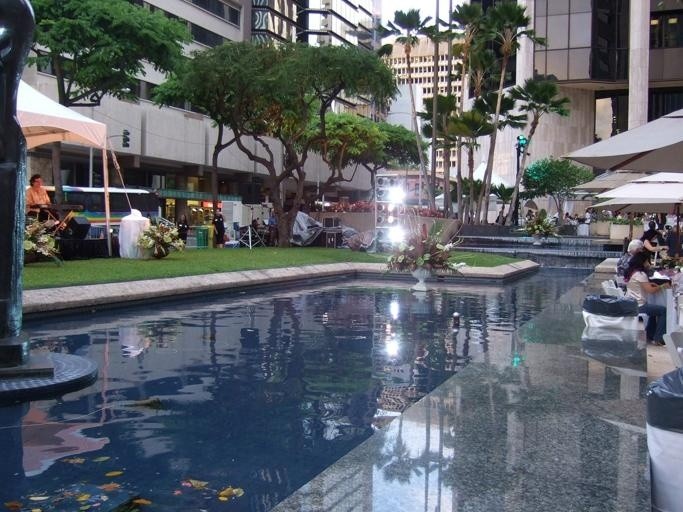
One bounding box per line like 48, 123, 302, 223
379, 207, 468, 279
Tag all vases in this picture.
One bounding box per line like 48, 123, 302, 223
408, 263, 435, 292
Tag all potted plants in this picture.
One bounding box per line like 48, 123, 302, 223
589, 210, 644, 242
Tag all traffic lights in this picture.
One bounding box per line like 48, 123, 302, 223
122, 130, 130, 147
517, 136, 528, 152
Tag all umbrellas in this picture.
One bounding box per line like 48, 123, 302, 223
593, 172, 683, 199
572, 170, 648, 190
560, 107, 683, 171
588, 198, 683, 267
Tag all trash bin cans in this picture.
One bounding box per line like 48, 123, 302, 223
195, 227, 208, 250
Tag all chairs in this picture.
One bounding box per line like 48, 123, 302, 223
603, 272, 649, 330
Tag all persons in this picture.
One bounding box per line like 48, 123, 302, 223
178, 215, 189, 243
26, 173, 59, 233
564, 208, 680, 346
212, 208, 225, 248
